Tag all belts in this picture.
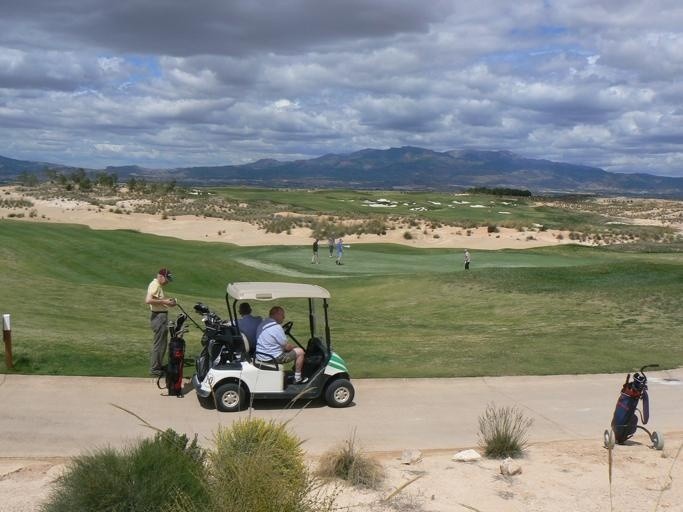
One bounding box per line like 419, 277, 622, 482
152, 311, 168, 313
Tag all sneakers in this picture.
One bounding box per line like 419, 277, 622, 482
151, 369, 167, 375
291, 376, 309, 384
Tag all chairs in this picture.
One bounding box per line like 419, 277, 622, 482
239, 331, 285, 393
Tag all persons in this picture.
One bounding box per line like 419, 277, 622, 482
334, 238, 343, 264
253, 306, 308, 384
222, 300, 262, 351
325, 237, 334, 258
462, 247, 471, 270
144, 267, 176, 375
310, 237, 321, 265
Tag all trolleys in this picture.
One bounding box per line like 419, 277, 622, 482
601, 362, 664, 452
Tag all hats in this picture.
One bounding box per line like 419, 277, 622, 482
158, 267, 174, 284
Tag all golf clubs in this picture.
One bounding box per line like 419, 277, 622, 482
167, 313, 189, 338
633, 373, 645, 389
193, 302, 222, 329
